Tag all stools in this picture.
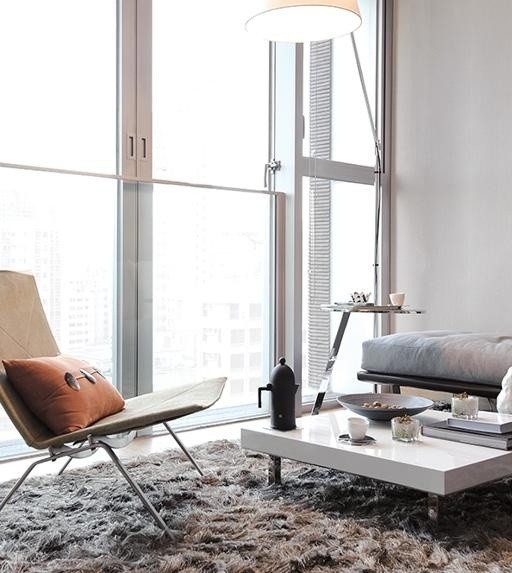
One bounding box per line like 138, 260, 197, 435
357, 331, 512, 413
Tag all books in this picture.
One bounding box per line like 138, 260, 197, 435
420, 414, 512, 451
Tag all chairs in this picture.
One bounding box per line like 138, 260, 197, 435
1, 271, 229, 545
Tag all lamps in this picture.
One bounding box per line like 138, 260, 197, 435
243, 1, 384, 394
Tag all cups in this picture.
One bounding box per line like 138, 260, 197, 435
390, 417, 421, 441
388, 291, 406, 306
346, 416, 371, 440
450, 395, 479, 420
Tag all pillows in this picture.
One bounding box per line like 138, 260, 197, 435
2, 353, 124, 436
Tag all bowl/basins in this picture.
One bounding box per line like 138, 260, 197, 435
334, 392, 436, 421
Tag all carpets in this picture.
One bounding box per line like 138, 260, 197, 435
1, 436, 512, 573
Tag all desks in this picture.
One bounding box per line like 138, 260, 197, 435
310, 305, 426, 416
